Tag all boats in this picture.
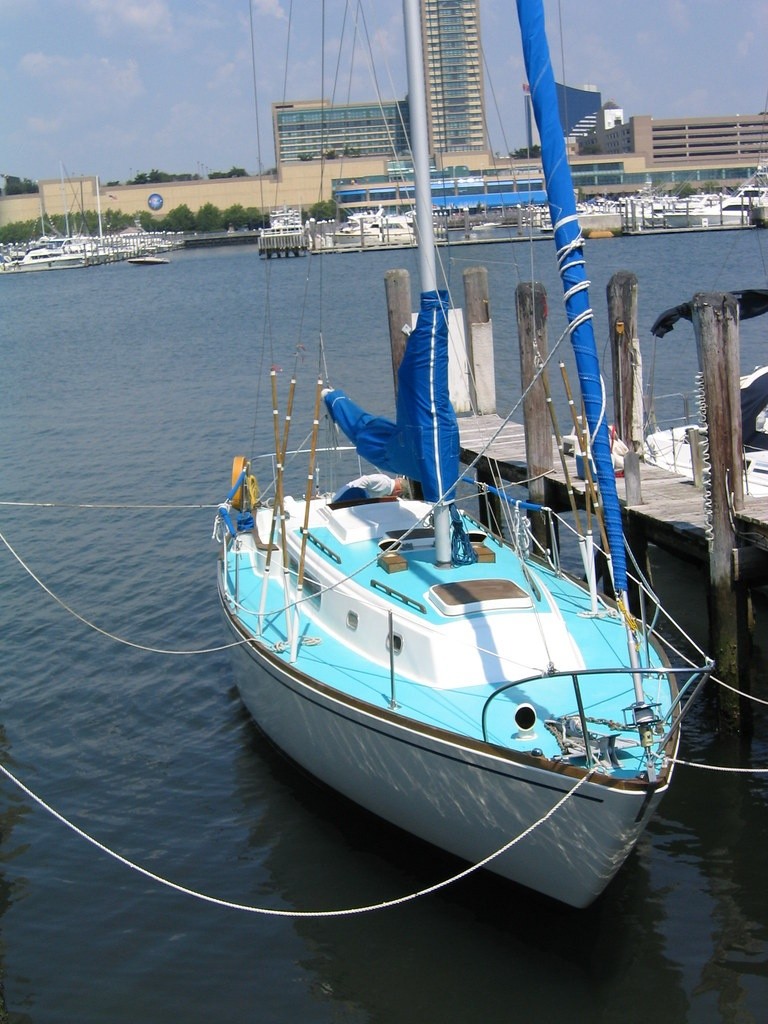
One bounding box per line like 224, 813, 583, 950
257, 198, 443, 260
0, 162, 178, 275
544, 167, 768, 240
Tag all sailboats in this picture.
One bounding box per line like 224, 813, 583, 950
214, 6, 720, 918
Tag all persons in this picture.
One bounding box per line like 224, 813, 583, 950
331, 474, 409, 505
